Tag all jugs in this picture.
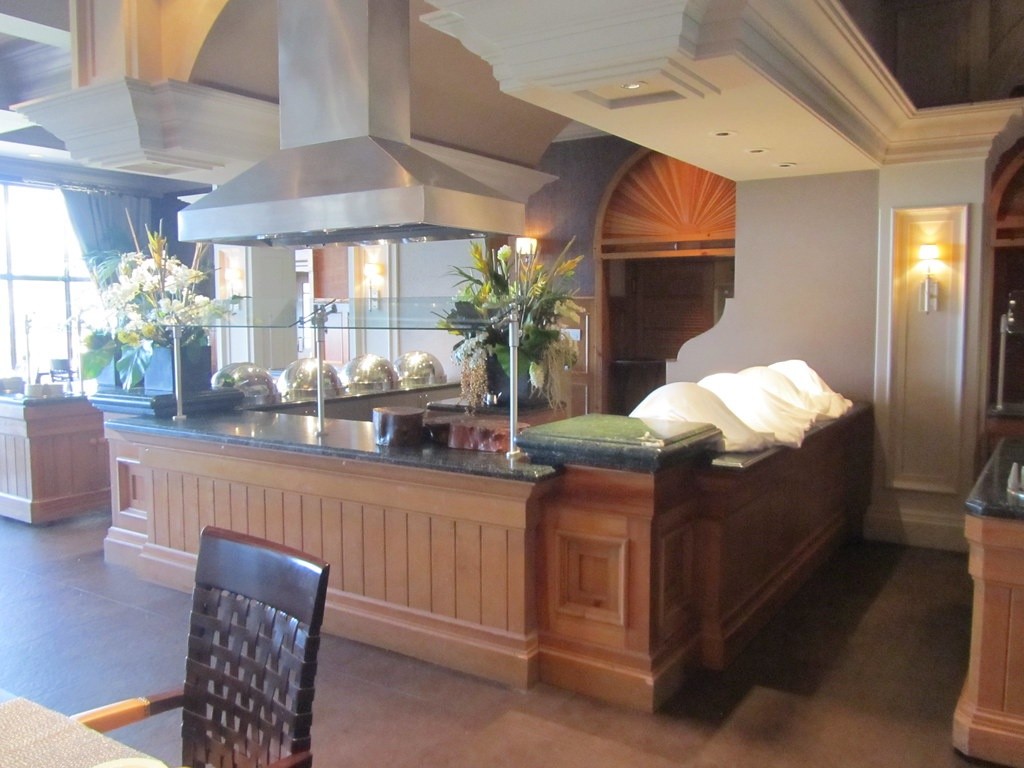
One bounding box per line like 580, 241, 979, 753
484, 391, 503, 405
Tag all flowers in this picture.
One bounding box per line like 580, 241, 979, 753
433, 234, 590, 412
74, 212, 254, 395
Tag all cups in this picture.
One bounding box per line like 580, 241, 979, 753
28, 385, 43, 398
48, 384, 63, 397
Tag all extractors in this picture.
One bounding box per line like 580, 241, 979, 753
178, 1, 526, 245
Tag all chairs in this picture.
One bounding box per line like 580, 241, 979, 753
68, 523, 332, 768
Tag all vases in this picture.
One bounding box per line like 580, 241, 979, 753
141, 346, 212, 400
486, 349, 552, 409
